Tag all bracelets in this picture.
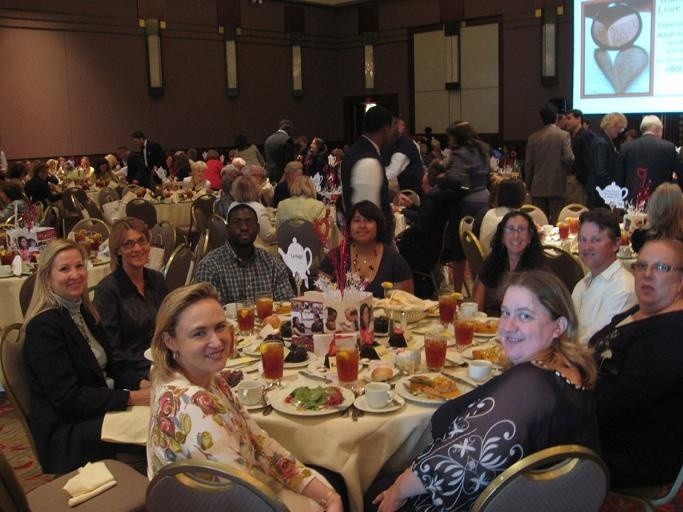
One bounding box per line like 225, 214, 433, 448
319, 489, 336, 507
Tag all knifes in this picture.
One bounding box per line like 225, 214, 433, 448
441, 371, 476, 389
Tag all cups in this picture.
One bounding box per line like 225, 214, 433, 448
618, 245, 630, 257
364, 383, 394, 408
224, 281, 478, 383
535, 218, 630, 247
468, 360, 492, 381
239, 381, 263, 404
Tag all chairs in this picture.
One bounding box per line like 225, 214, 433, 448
0, 154, 683, 512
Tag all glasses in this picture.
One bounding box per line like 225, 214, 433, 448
504, 227, 529, 233
630, 261, 683, 272
121, 236, 147, 250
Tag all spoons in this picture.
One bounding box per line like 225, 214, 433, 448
350, 385, 367, 420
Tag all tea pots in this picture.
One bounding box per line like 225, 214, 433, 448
327, 154, 336, 165
595, 180, 628, 205
277, 237, 312, 277
490, 156, 499, 169
310, 171, 323, 186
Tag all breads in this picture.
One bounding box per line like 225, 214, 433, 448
263, 315, 281, 330
370, 366, 393, 381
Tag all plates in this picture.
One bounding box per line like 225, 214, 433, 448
473, 317, 501, 337
0, 255, 110, 277
267, 385, 355, 414
352, 394, 405, 414
142, 346, 154, 361
462, 345, 503, 368
616, 254, 637, 259
396, 371, 475, 404
234, 387, 271, 409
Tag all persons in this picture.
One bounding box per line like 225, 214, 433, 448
569, 206, 640, 350
145, 279, 343, 512
92, 217, 169, 391
20, 237, 150, 477
470, 209, 570, 318
371, 264, 602, 512
0, 105, 681, 306
582, 233, 681, 485
